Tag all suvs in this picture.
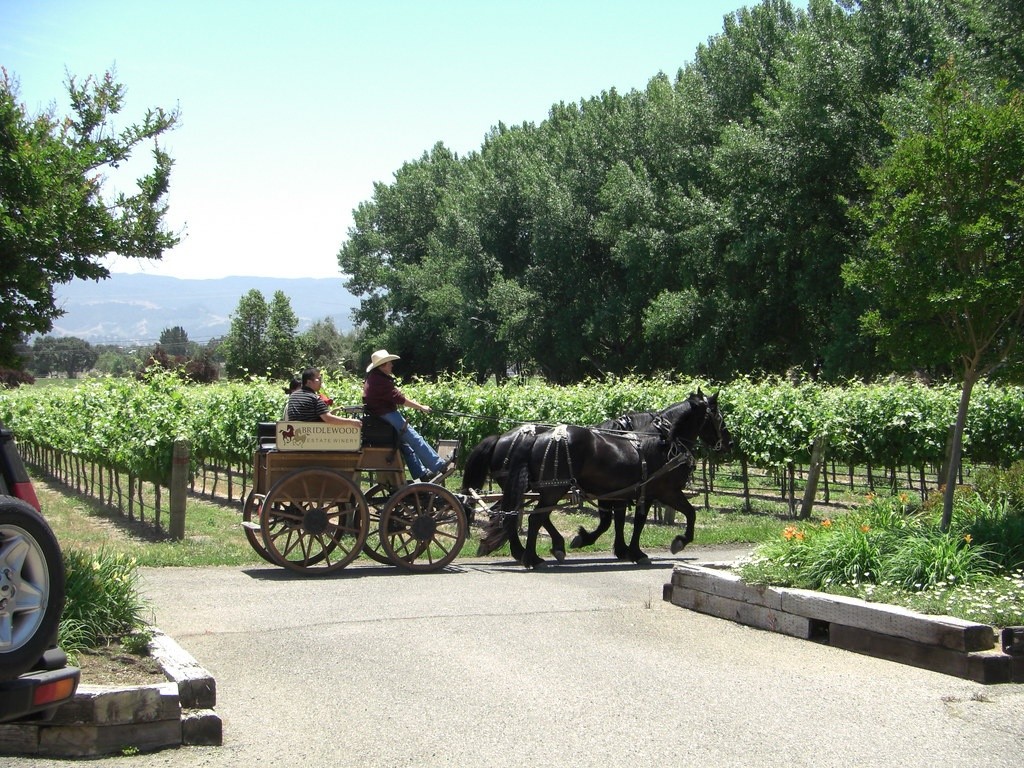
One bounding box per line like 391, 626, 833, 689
0, 428, 81, 724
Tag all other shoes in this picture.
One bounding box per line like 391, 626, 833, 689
420, 470, 436, 482
440, 456, 451, 475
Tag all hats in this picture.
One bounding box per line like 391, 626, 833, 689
366, 350, 400, 373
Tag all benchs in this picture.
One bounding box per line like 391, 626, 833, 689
341, 405, 397, 447
256, 420, 362, 453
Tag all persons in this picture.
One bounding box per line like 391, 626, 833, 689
282, 367, 362, 429
362, 349, 453, 483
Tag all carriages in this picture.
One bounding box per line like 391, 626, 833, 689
241, 386, 735, 575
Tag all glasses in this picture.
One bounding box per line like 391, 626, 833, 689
310, 374, 324, 383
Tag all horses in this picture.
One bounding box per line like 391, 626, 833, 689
461, 385, 735, 570
